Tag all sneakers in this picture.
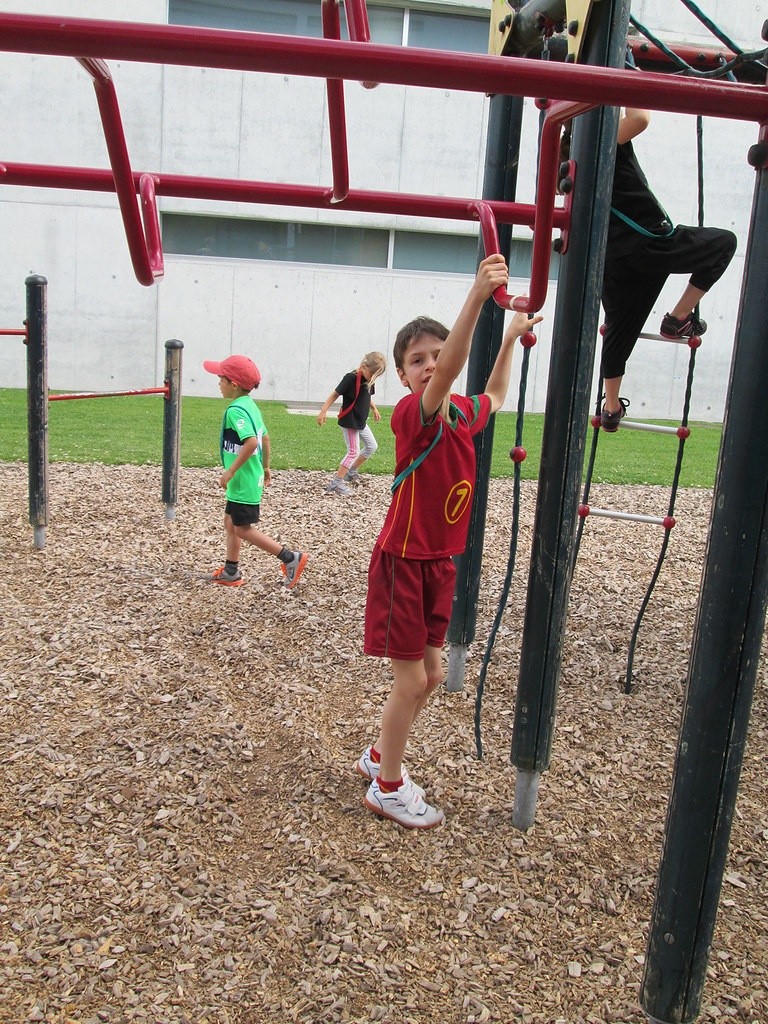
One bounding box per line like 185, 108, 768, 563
363, 776, 444, 830
202, 566, 244, 586
602, 397, 630, 432
326, 480, 354, 497
660, 312, 708, 338
356, 747, 427, 801
281, 551, 308, 588
343, 470, 362, 481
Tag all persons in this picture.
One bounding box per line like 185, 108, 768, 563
355, 251, 544, 827
201, 354, 309, 588
542, 28, 737, 434
318, 351, 388, 492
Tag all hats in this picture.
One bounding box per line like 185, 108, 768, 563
203, 355, 261, 391
